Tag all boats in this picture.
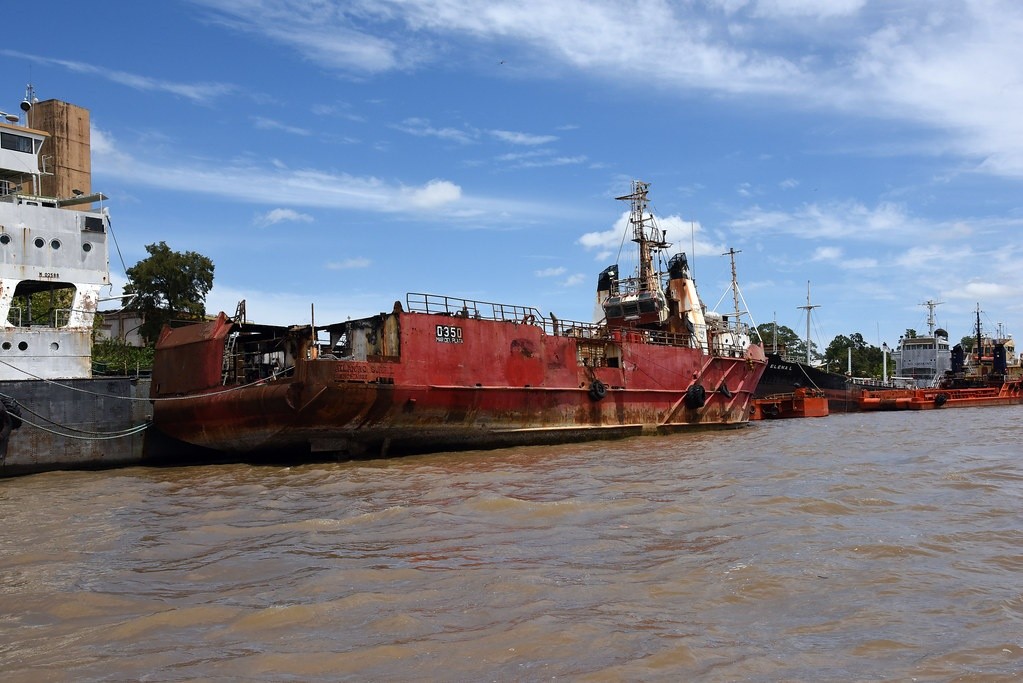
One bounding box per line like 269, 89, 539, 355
855, 303, 1023, 410
746, 277, 912, 422
149, 179, 770, 461
0, 81, 157, 471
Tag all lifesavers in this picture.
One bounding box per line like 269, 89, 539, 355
722, 384, 732, 398
686, 385, 706, 407
590, 379, 606, 401
749, 405, 756, 414
